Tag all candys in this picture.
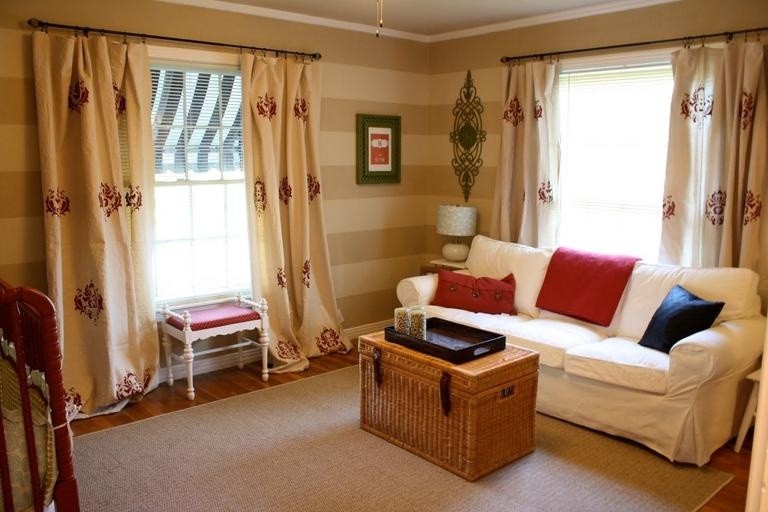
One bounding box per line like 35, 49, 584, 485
410, 313, 426, 340
395, 308, 410, 335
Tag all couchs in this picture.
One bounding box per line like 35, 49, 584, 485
394, 233, 766, 469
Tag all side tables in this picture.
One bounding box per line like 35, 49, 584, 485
429, 258, 468, 271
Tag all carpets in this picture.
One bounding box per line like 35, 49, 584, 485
71, 362, 737, 512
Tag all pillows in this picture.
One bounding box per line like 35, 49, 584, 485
429, 269, 518, 317
637, 283, 727, 355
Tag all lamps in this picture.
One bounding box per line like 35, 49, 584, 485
435, 203, 480, 264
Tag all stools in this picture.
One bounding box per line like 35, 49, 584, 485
160, 290, 272, 402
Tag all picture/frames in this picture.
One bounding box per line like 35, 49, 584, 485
354, 110, 403, 187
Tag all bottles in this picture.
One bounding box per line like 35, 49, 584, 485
393, 306, 411, 336
410, 306, 426, 340
